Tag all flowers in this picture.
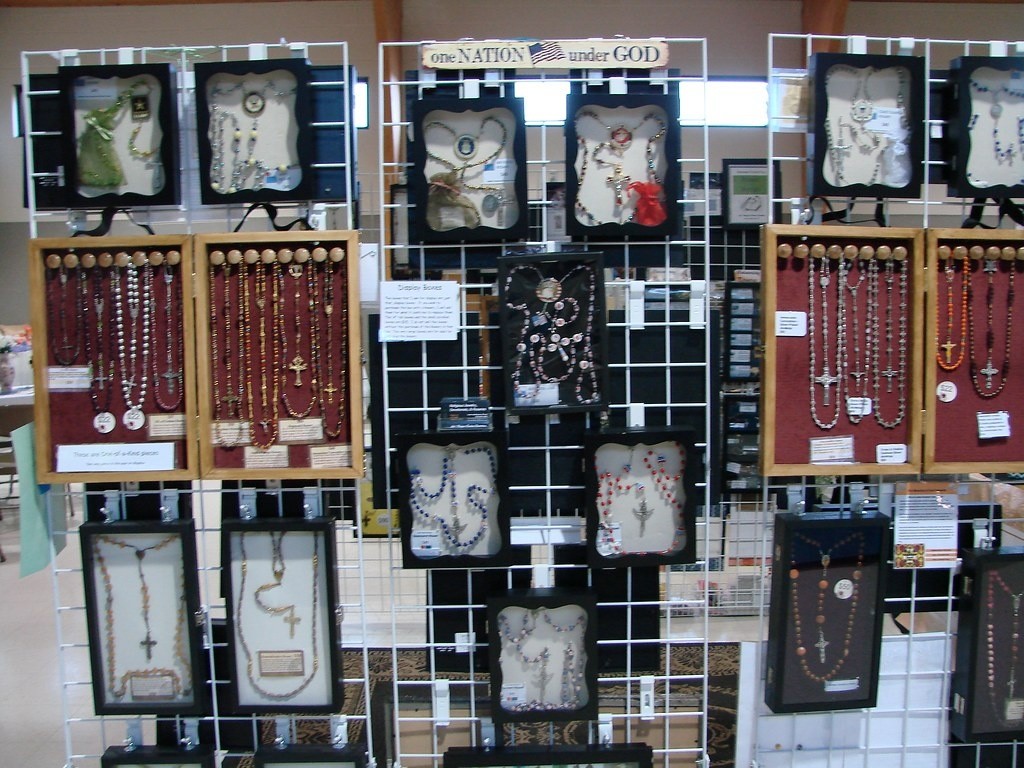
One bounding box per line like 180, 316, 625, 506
0, 332, 18, 355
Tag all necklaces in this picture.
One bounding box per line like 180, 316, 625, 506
969, 72, 1024, 189
824, 67, 910, 186
503, 263, 601, 405
92, 532, 192, 700
76, 78, 162, 189
496, 607, 588, 711
210, 75, 298, 193
936, 254, 1016, 398
44, 254, 184, 435
411, 446, 496, 552
236, 529, 319, 702
421, 115, 507, 227
210, 256, 346, 447
988, 569, 1023, 730
593, 441, 682, 556
789, 531, 867, 685
809, 254, 906, 429
574, 111, 666, 221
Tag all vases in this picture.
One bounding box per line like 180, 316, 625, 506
0, 354, 15, 395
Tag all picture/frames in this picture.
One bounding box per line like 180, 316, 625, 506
722, 157, 779, 229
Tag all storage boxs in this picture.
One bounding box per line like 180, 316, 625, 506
192, 56, 314, 204
502, 251, 609, 415
61, 64, 180, 207
422, 97, 532, 243
218, 518, 344, 710
948, 544, 1024, 744
808, 53, 924, 200
79, 519, 208, 715
488, 587, 599, 723
763, 509, 891, 716
565, 92, 682, 242
947, 56, 1024, 200
393, 426, 515, 568
584, 423, 697, 568
